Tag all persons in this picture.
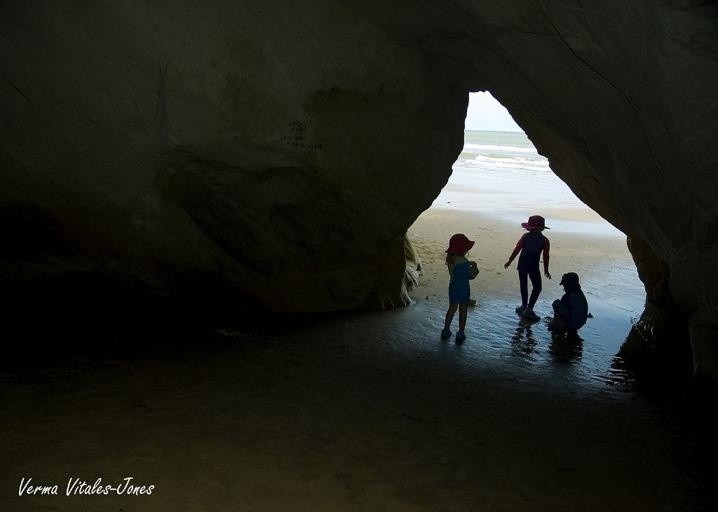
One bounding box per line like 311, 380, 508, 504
442, 234, 479, 341
504, 216, 551, 320
548, 273, 588, 346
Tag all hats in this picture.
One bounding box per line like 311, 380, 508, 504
521, 215, 550, 230
559, 272, 579, 285
445, 233, 474, 255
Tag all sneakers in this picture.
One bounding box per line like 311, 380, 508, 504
456, 332, 466, 339
515, 305, 540, 318
441, 330, 452, 338
